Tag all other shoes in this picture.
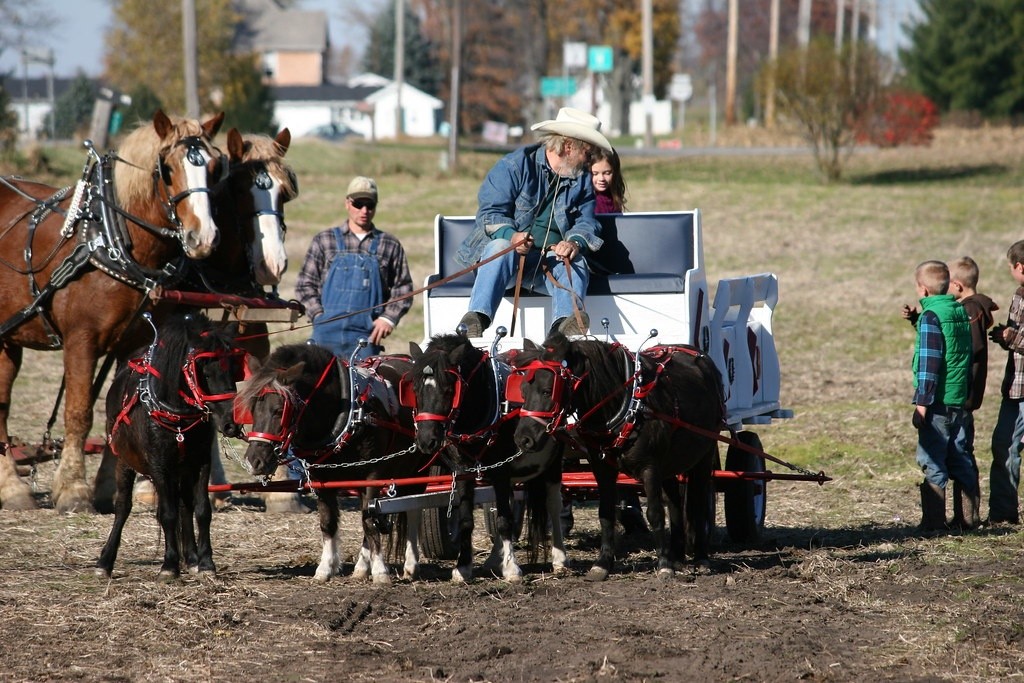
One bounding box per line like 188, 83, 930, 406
558, 312, 590, 335
460, 311, 483, 338
910, 517, 1019, 537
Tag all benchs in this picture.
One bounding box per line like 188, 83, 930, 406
424, 208, 703, 297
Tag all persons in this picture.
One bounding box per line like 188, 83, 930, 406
983, 239, 1024, 524
285, 176, 414, 482
588, 145, 628, 214
453, 107, 614, 337
945, 255, 999, 474
901, 261, 976, 534
136, 432, 233, 511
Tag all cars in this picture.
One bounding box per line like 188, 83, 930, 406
303, 123, 365, 142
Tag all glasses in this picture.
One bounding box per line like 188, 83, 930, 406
348, 196, 376, 210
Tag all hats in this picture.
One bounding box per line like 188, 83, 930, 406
530, 107, 613, 155
347, 176, 377, 201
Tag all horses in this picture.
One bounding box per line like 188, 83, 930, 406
235, 317, 727, 587
0, 105, 302, 583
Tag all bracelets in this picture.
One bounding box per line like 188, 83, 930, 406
570, 239, 580, 248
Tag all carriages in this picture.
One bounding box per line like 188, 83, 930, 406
94, 207, 834, 589
